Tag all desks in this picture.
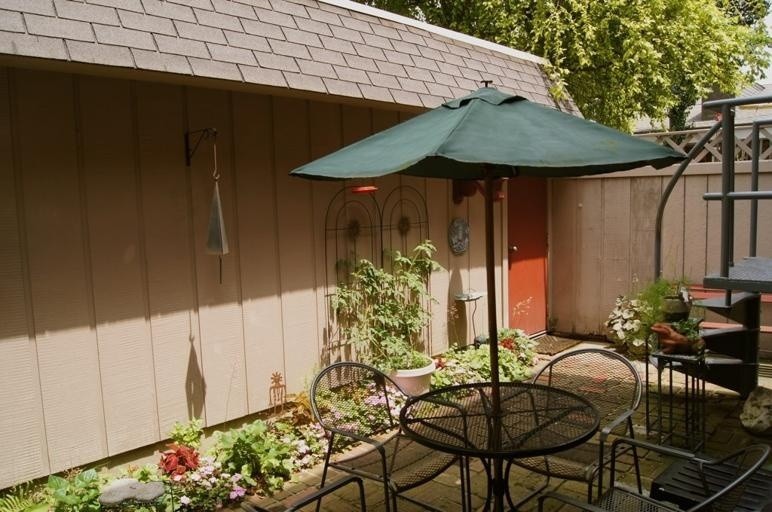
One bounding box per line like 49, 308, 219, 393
383, 381, 606, 512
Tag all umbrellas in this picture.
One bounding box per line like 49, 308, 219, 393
289, 78, 692, 512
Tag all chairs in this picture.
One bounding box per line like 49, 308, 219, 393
307, 360, 470, 512
503, 349, 644, 512
535, 436, 771, 512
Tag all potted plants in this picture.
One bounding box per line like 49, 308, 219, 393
324, 240, 445, 401
650, 274, 712, 357
